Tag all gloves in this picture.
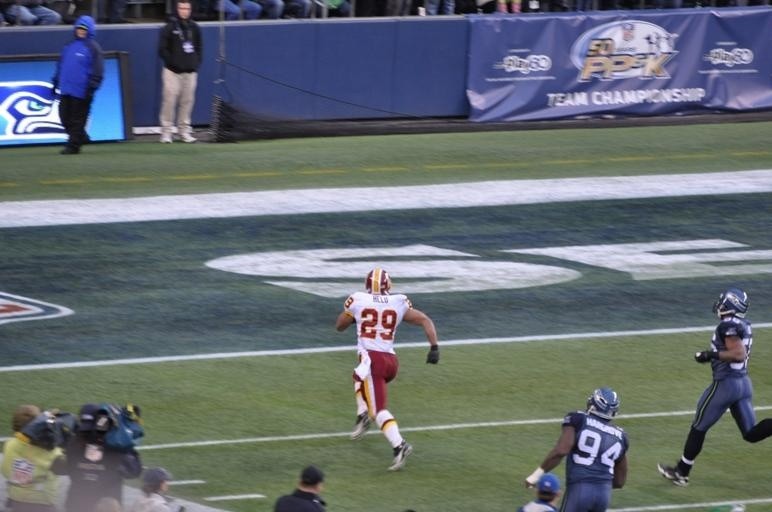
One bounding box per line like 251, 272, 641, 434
695, 351, 710, 363
426, 351, 439, 364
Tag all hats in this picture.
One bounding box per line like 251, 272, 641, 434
79, 404, 99, 432
536, 472, 560, 493
302, 465, 324, 485
143, 468, 173, 481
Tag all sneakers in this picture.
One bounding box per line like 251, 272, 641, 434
387, 443, 414, 471
61, 147, 78, 154
657, 463, 688, 487
160, 133, 173, 144
349, 414, 372, 441
180, 133, 198, 143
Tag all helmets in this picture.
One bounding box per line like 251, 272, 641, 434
366, 267, 391, 295
712, 287, 751, 319
586, 386, 620, 420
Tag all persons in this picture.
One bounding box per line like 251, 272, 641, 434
1, 1, 772, 29
516, 472, 564, 511
50, 14, 106, 155
525, 385, 630, 511
65, 402, 141, 512
96, 496, 121, 512
154, 1, 203, 144
273, 463, 329, 511
655, 286, 772, 487
336, 267, 442, 476
1, 402, 70, 512
129, 466, 177, 512
